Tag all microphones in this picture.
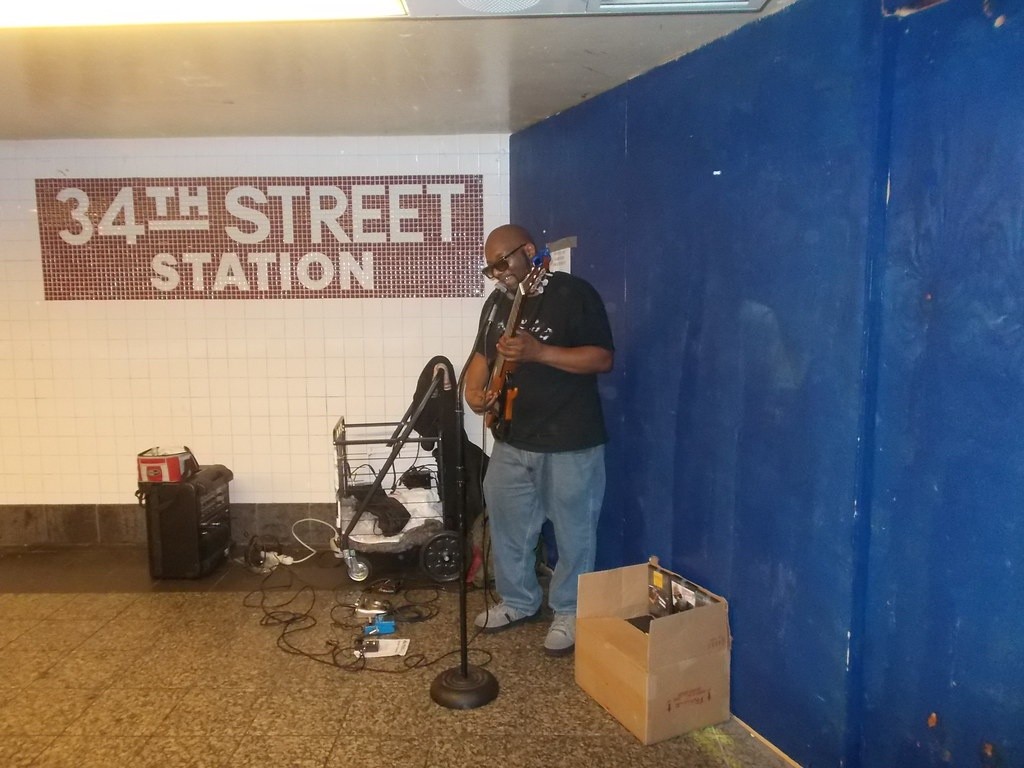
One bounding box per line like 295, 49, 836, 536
485, 280, 508, 333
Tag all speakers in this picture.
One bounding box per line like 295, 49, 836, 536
145, 463, 234, 580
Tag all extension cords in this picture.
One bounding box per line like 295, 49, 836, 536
260, 551, 293, 565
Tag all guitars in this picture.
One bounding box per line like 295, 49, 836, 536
486, 251, 552, 444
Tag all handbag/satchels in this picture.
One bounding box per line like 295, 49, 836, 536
371, 497, 412, 536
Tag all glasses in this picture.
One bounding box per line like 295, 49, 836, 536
482, 243, 526, 278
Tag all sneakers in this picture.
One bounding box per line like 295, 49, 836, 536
475, 602, 542, 634
543, 610, 578, 656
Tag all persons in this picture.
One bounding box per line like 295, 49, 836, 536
464, 225, 614, 657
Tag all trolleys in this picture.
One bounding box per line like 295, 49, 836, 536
329, 367, 475, 585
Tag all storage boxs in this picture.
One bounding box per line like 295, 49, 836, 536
571, 556, 730, 749
136, 446, 200, 482
